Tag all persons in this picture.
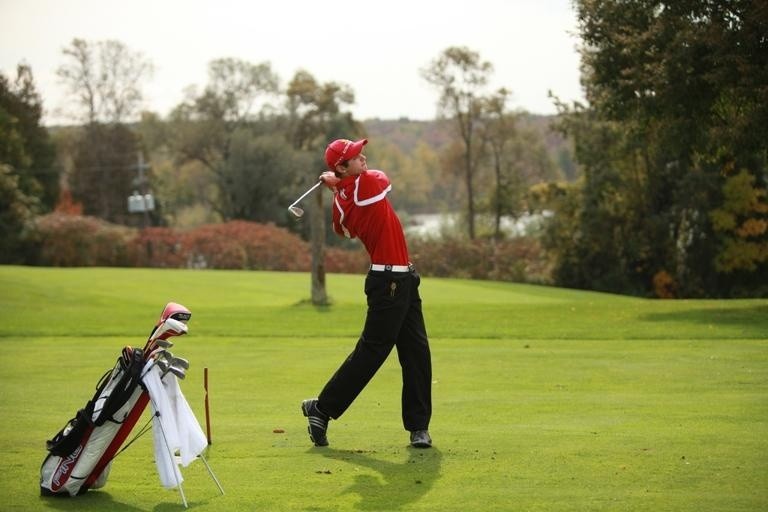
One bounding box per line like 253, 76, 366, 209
301, 138, 434, 449
142, 188, 162, 227
127, 189, 147, 226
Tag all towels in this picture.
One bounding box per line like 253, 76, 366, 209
140, 358, 208, 488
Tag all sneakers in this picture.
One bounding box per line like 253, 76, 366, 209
301, 399, 329, 446
410, 429, 431, 447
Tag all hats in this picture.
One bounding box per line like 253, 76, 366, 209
325, 139, 368, 173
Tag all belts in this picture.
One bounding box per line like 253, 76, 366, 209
369, 261, 416, 273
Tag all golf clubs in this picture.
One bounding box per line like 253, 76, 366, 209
139, 302, 191, 381
287, 179, 325, 222
